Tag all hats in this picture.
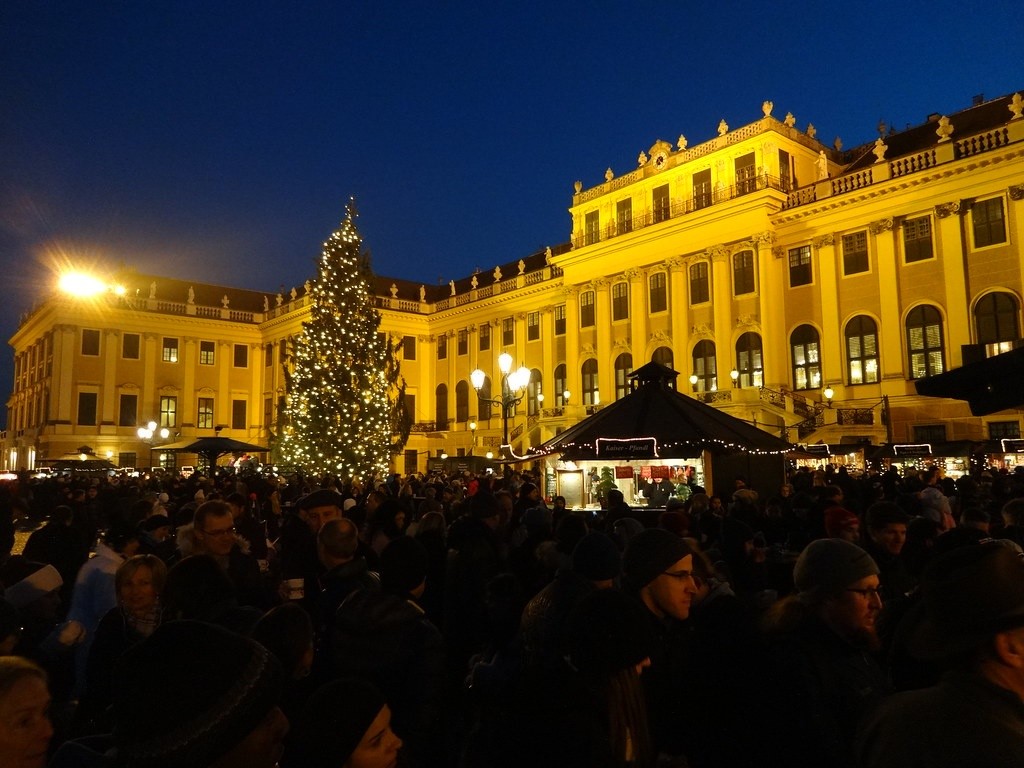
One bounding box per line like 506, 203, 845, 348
111, 620, 280, 767
623, 527, 692, 589
471, 495, 499, 518
569, 533, 620, 580
934, 539, 1024, 635
518, 506, 552, 529
793, 539, 880, 597
300, 677, 387, 768
825, 506, 860, 538
658, 512, 688, 531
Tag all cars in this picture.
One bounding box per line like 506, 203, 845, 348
179, 466, 194, 477
0, 470, 18, 480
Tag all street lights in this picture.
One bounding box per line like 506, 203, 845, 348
138, 422, 169, 478
470, 353, 530, 445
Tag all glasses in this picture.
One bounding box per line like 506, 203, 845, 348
842, 585, 882, 599
201, 525, 237, 537
663, 570, 696, 583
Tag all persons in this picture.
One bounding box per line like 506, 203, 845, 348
0, 458, 1024, 768
